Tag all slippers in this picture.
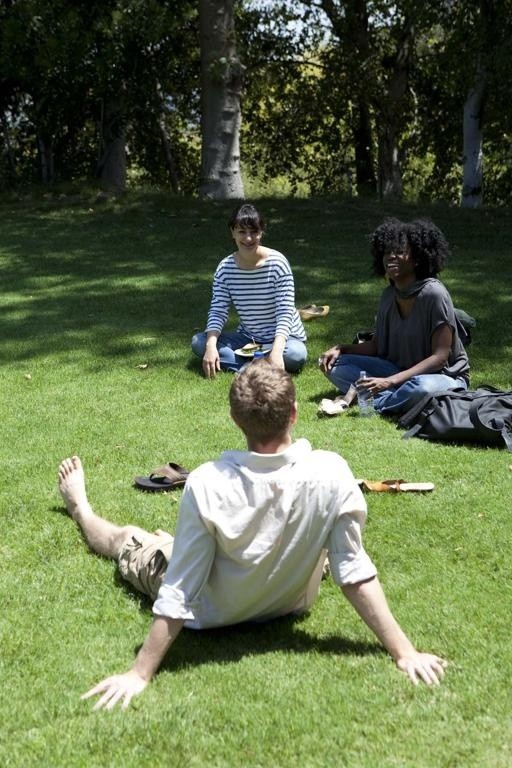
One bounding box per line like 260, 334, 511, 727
135, 462, 189, 490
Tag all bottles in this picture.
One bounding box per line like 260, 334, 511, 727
356, 370, 375, 418
250, 352, 264, 364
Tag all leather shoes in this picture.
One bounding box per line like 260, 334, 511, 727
298, 304, 329, 321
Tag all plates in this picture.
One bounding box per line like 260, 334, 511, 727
234, 348, 272, 358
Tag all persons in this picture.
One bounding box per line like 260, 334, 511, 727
56, 360, 452, 712
315, 216, 471, 414
190, 201, 310, 380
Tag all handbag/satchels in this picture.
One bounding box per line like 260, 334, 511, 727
398, 384, 512, 451
